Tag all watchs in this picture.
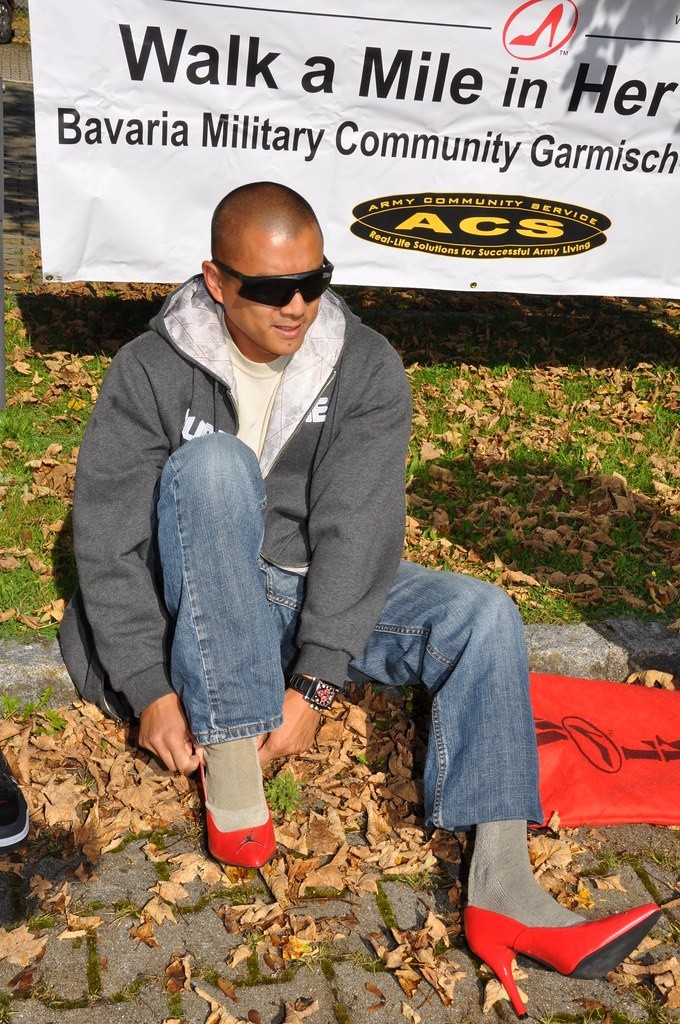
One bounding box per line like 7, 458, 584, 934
290, 674, 338, 712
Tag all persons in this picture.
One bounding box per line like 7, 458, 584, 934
57, 182, 664, 1021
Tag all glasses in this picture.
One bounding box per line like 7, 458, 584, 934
210, 255, 333, 306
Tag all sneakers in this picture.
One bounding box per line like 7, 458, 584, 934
0, 747, 31, 852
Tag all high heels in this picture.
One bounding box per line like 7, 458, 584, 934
199, 761, 278, 868
462, 901, 664, 1018
